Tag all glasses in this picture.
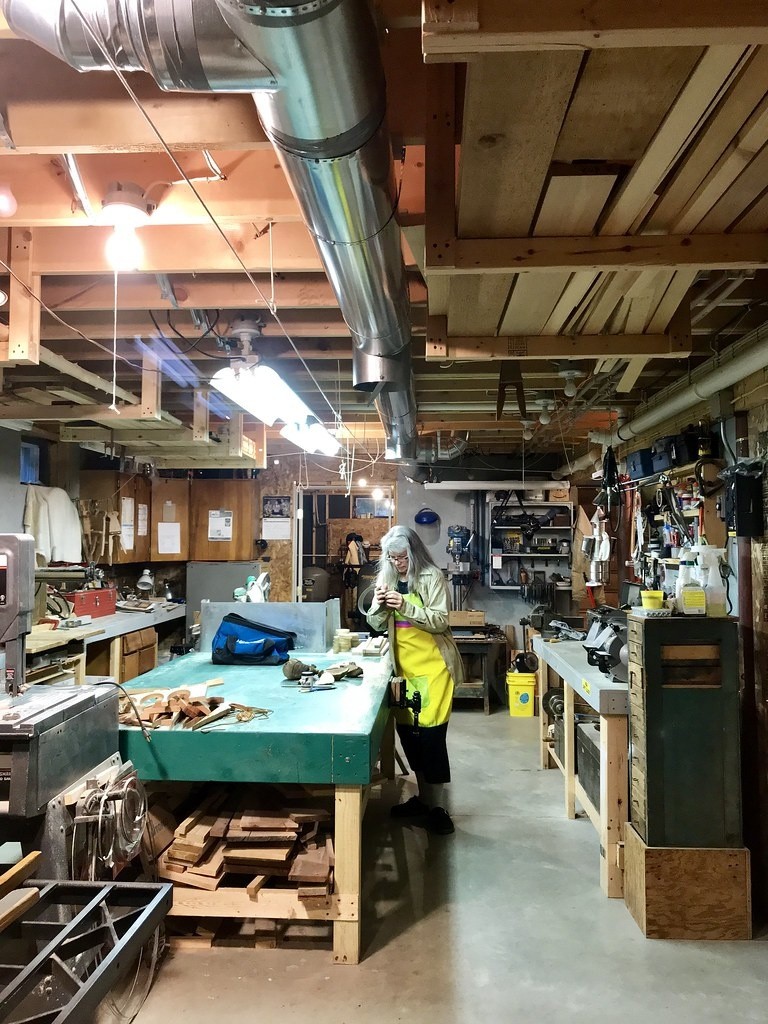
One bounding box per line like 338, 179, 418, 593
385, 554, 408, 562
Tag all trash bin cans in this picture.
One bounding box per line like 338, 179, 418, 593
506, 670, 536, 717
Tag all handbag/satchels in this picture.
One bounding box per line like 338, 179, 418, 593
211, 613, 297, 665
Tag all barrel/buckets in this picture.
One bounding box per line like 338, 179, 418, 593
505, 672, 536, 717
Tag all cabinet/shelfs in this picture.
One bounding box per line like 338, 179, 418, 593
532, 635, 629, 898
81, 470, 260, 564
625, 457, 727, 566
623, 614, 753, 942
490, 501, 574, 590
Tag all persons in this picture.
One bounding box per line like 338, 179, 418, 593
366, 524, 463, 835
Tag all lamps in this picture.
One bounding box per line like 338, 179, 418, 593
137, 569, 156, 599
210, 316, 352, 457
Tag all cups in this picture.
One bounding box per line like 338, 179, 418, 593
639, 591, 665, 609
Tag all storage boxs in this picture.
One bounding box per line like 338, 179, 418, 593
554, 716, 600, 775
86, 627, 158, 683
576, 723, 600, 814
449, 611, 486, 627
64, 588, 117, 618
549, 489, 569, 501
603, 555, 618, 607
511, 629, 560, 688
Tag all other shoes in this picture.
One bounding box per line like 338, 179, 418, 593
391, 795, 431, 817
426, 807, 455, 835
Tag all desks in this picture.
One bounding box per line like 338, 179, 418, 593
119, 636, 398, 965
25, 602, 186, 685
453, 639, 493, 716
